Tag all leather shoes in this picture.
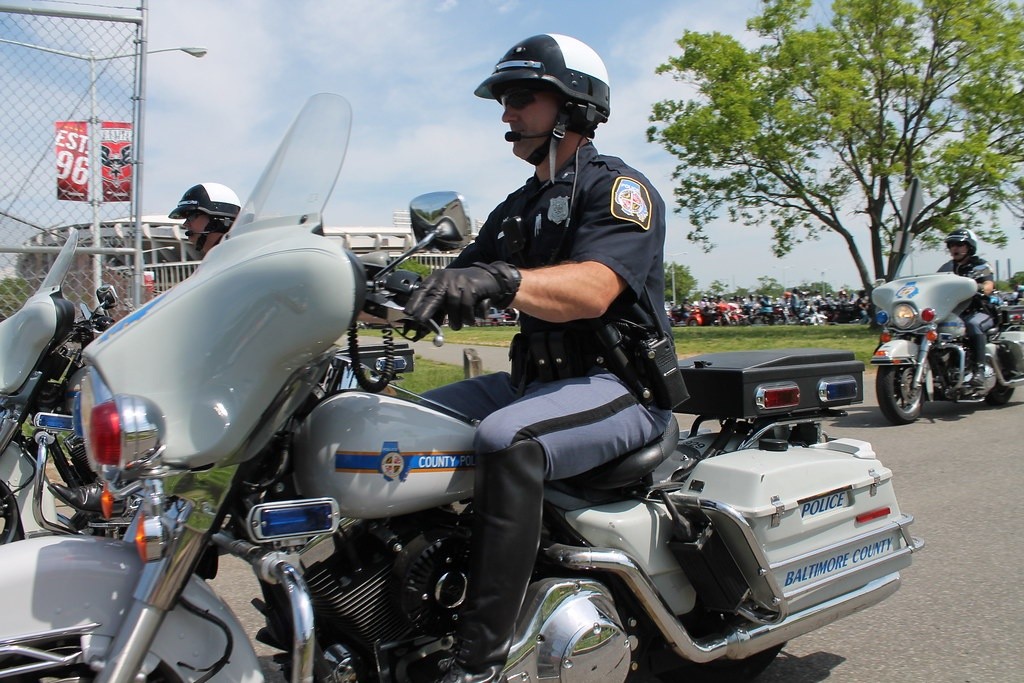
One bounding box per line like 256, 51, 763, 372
48, 479, 126, 516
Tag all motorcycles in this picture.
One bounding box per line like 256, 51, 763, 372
865, 240, 1024, 423
0, 223, 417, 545
0, 91, 928, 683
667, 296, 872, 327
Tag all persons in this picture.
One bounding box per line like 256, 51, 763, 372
936, 228, 1001, 388
354, 32, 691, 683
1003, 284, 1024, 305
46, 181, 242, 513
668, 286, 871, 327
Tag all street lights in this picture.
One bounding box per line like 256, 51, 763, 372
0, 40, 208, 317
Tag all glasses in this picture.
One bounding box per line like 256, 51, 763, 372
187, 213, 205, 221
950, 242, 966, 247
499, 86, 554, 109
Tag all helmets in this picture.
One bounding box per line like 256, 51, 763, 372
473, 33, 610, 128
169, 182, 239, 221
682, 286, 865, 305
945, 228, 977, 255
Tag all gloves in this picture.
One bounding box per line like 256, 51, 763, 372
403, 261, 516, 332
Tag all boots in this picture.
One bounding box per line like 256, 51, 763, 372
257, 627, 284, 649
435, 439, 546, 683
969, 333, 987, 386
